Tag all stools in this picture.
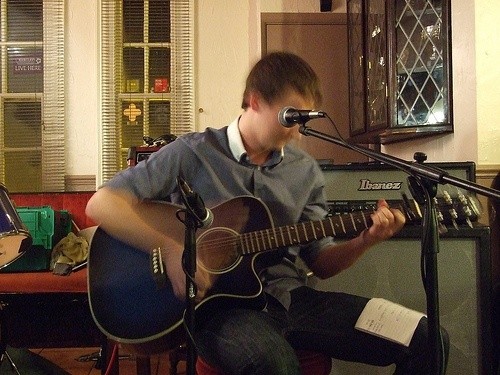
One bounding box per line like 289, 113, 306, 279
196, 348, 332, 375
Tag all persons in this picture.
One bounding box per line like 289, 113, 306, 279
84, 51, 451, 375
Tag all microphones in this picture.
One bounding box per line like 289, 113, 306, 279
278, 106, 326, 128
176, 176, 215, 230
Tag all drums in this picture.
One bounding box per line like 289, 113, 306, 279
0, 182, 34, 270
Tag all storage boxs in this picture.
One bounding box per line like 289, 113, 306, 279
0, 206, 73, 272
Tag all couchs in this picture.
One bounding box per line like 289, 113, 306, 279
0, 191, 152, 375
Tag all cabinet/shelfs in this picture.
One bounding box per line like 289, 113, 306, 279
347, 0, 455, 145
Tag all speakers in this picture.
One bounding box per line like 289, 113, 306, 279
295, 227, 494, 375
0, 344, 70, 375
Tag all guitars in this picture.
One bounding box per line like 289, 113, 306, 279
86, 187, 486, 356
